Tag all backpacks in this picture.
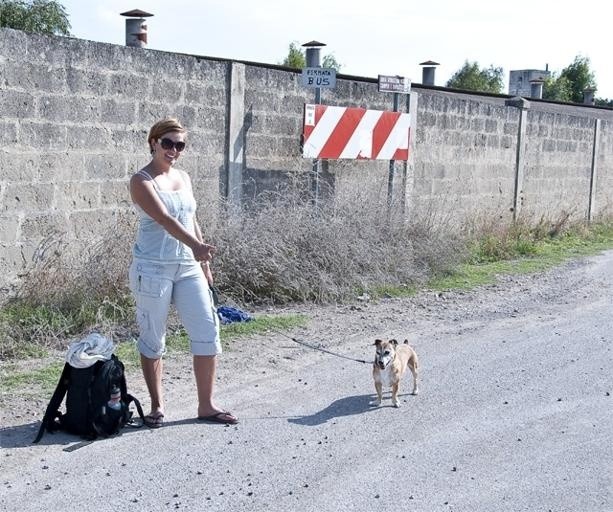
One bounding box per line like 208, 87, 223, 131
44, 353, 133, 440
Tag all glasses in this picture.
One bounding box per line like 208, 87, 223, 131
157, 137, 185, 151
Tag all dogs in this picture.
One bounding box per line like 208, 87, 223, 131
369, 339, 419, 408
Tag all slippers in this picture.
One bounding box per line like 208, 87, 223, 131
198, 409, 240, 424
143, 415, 164, 428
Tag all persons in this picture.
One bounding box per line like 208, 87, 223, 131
128, 118, 241, 428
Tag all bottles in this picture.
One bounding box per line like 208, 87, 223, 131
110, 384, 121, 403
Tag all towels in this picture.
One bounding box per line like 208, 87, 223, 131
63, 332, 117, 369
